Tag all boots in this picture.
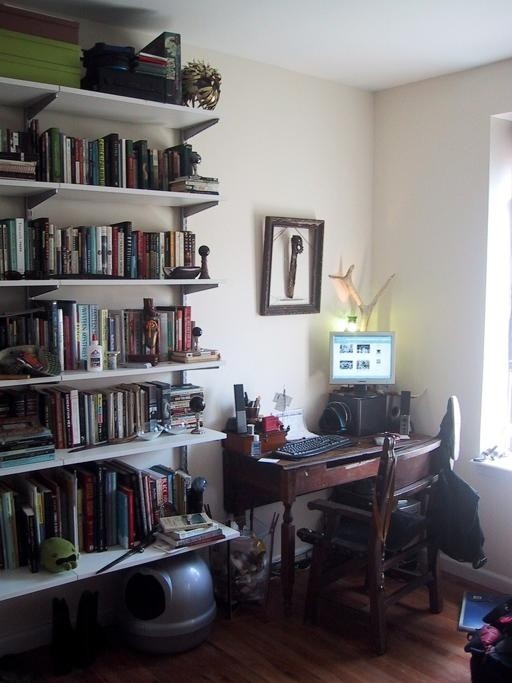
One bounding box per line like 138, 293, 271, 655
49, 590, 110, 678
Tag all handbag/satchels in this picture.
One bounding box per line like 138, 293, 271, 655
463, 597, 512, 683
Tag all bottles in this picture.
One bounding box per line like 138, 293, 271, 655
86, 335, 102, 373
251, 435, 261, 458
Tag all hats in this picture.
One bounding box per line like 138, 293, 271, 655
0, 344, 61, 377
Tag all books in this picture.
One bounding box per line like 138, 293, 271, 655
0, 383, 206, 468
1, 458, 226, 574
1, 297, 221, 371
1, 114, 220, 195
2, 217, 197, 279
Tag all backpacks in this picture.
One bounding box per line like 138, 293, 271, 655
433, 433, 487, 570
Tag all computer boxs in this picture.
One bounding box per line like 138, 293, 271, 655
341, 486, 421, 577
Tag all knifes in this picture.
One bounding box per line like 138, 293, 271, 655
96, 535, 157, 574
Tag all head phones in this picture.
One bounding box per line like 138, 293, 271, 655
323, 401, 351, 434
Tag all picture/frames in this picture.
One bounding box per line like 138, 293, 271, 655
259, 215, 325, 316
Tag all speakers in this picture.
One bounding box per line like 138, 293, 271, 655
399, 391, 410, 434
329, 390, 390, 436
234, 384, 247, 433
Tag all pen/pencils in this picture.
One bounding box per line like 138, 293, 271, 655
244, 391, 260, 407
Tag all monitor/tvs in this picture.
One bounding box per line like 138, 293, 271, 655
328, 331, 396, 397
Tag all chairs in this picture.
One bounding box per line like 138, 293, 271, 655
295, 433, 442, 656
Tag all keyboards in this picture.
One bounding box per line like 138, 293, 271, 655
273, 433, 351, 460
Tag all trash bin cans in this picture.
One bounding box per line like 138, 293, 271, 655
203, 515, 280, 613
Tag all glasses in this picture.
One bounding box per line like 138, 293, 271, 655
2, 269, 44, 280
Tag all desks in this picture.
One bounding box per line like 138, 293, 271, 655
222, 429, 441, 611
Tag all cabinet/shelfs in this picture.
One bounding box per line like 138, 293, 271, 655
0, 73, 241, 603
